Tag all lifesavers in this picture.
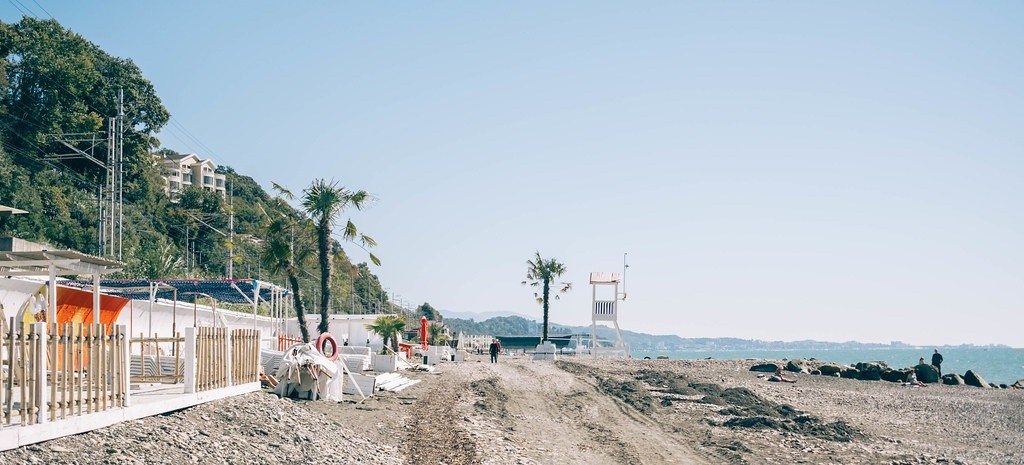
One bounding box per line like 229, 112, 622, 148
316, 332, 340, 362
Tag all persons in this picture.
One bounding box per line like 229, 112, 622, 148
775, 364, 798, 384
366, 338, 371, 347
495, 339, 501, 358
343, 338, 349, 346
932, 348, 944, 380
897, 356, 929, 388
489, 338, 498, 364
